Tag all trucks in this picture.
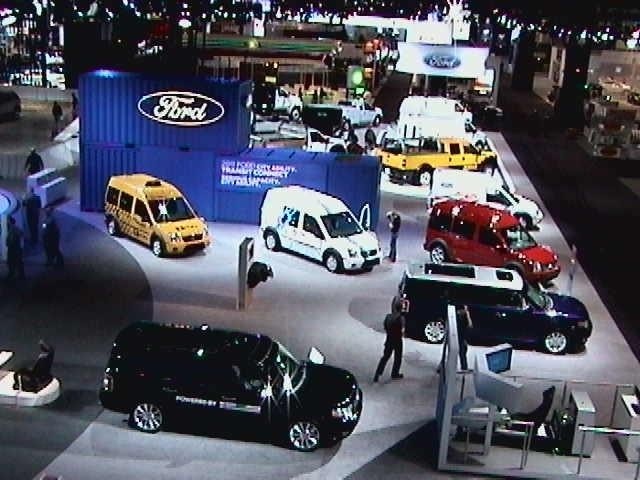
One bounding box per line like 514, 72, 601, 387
274, 86, 302, 122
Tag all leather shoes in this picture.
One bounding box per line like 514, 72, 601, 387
393, 374, 403, 378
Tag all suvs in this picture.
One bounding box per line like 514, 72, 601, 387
395, 263, 592, 354
0, 86, 21, 119
98, 322, 363, 448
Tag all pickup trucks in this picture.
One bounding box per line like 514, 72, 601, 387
265, 124, 348, 153
382, 136, 498, 186
337, 98, 384, 130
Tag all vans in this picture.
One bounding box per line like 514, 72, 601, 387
104, 173, 213, 258
401, 94, 477, 128
432, 168, 544, 231
261, 183, 381, 273
426, 202, 562, 287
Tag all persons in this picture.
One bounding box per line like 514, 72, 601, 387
5, 218, 26, 282
387, 212, 401, 262
70, 92, 78, 115
352, 96, 365, 110
53, 101, 62, 131
40, 208, 61, 265
331, 117, 378, 157
24, 147, 45, 174
372, 300, 404, 383
22, 187, 42, 241
451, 304, 475, 374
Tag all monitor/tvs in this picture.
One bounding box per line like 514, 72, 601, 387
487, 347, 512, 373
